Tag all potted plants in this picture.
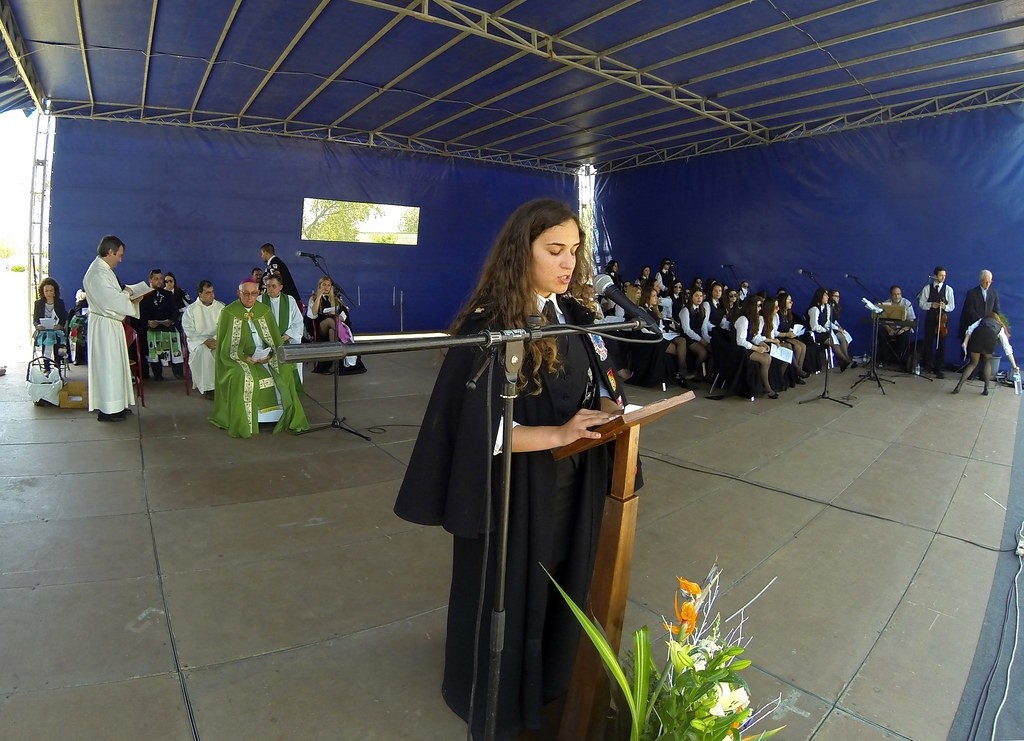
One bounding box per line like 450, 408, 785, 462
981, 313, 1010, 380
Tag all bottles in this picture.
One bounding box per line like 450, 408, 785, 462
1013, 371, 1022, 394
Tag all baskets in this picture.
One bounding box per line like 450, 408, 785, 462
26, 356, 64, 408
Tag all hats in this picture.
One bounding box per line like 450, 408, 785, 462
241, 278, 257, 284
661, 258, 673, 267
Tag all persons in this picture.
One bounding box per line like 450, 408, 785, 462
878, 285, 917, 362
918, 266, 955, 373
394, 198, 645, 741
594, 256, 859, 398
952, 269, 1020, 396
33, 235, 369, 440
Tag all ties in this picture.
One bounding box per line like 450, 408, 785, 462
935, 285, 939, 293
546, 299, 569, 358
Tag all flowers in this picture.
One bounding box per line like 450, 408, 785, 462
539, 554, 785, 741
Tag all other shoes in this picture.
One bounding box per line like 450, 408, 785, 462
679, 373, 695, 381
841, 361, 858, 372
768, 370, 810, 399
983, 388, 988, 394
682, 381, 699, 390
98, 409, 132, 421
174, 370, 185, 380
154, 374, 164, 382
952, 383, 961, 393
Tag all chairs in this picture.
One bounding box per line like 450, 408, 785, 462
32, 311, 71, 371
184, 333, 191, 394
296, 300, 317, 369
621, 312, 916, 401
121, 322, 145, 407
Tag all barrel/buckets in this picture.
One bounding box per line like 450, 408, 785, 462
991, 354, 1002, 375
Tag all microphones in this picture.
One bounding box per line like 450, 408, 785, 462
845, 273, 859, 279
296, 251, 323, 258
929, 276, 938, 279
593, 274, 663, 337
798, 269, 816, 275
720, 265, 734, 268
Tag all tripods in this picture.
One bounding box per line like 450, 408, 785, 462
295, 258, 370, 441
798, 275, 934, 408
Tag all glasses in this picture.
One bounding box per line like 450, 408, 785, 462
697, 281, 702, 284
240, 290, 259, 296
730, 294, 737, 298
674, 285, 682, 288
744, 286, 749, 288
164, 279, 173, 283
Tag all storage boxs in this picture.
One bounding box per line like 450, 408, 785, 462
59, 381, 88, 408
